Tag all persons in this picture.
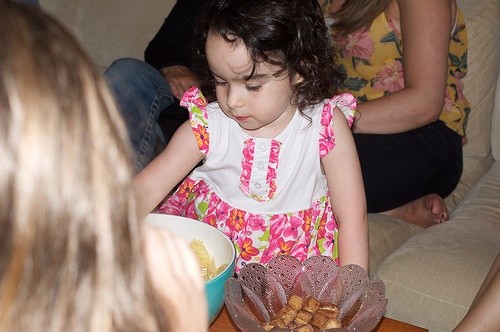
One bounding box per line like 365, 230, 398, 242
447, 253, 500, 332
0, 0, 209, 332
98, 0, 241, 178
102, 0, 369, 297
264, 0, 471, 230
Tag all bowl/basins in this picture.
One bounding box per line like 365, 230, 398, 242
144, 213, 236, 327
225, 256, 389, 332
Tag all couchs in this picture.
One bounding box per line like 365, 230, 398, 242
40, 0, 500, 332
